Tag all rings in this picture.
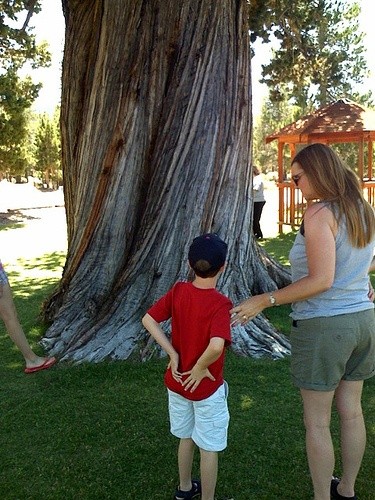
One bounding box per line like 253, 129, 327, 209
243, 315, 248, 321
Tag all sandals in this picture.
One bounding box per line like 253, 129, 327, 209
331, 475, 357, 500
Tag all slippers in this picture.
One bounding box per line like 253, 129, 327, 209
24, 356, 56, 374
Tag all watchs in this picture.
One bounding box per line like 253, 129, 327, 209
269, 291, 279, 306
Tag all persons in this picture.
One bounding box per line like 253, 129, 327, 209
230, 143, 375, 500
252, 166, 266, 239
0, 261, 56, 372
142, 233, 234, 500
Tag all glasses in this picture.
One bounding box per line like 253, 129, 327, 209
293, 171, 305, 186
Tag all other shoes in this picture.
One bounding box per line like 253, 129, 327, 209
174, 481, 202, 500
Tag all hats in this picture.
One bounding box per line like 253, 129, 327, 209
186, 233, 228, 277
